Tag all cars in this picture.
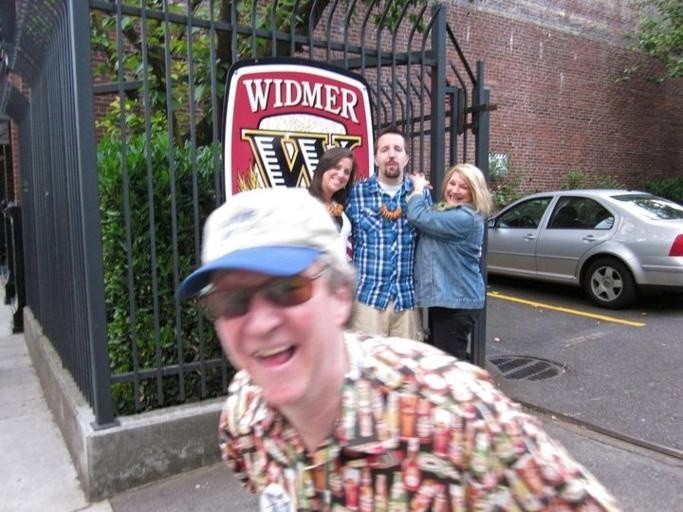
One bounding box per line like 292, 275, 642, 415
485, 187, 683, 309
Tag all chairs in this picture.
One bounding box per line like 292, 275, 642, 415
554, 205, 609, 230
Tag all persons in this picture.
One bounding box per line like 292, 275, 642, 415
175, 184, 620, 511
344, 123, 432, 346
308, 145, 358, 262
401, 160, 494, 366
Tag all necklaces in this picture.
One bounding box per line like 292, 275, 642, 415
319, 194, 344, 216
377, 180, 404, 223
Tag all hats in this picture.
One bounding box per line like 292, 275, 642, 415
174, 185, 343, 303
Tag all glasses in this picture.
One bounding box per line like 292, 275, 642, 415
193, 261, 330, 322
